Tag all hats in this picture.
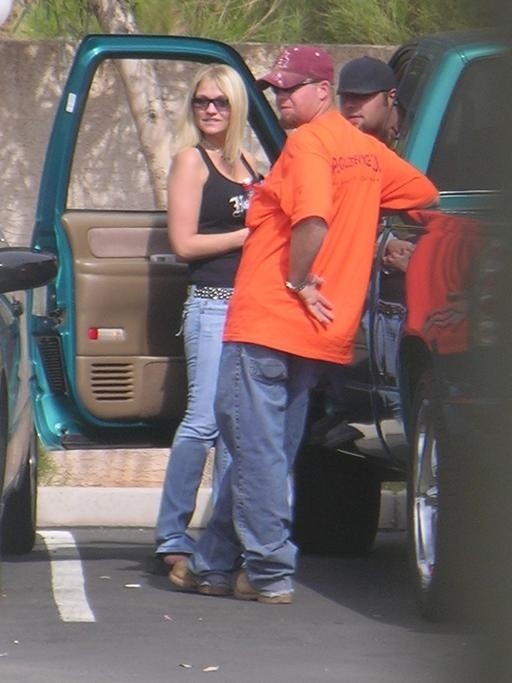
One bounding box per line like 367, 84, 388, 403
254, 42, 334, 91
335, 57, 398, 94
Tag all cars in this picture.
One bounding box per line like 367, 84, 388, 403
0, 226, 64, 554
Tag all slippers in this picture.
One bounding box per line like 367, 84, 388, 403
142, 549, 191, 577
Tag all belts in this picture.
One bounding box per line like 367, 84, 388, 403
187, 285, 231, 301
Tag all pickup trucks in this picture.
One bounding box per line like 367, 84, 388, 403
26, 27, 511, 601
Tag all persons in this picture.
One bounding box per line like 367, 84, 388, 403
337, 53, 397, 136
397, 209, 485, 458
155, 61, 265, 567
167, 43, 440, 606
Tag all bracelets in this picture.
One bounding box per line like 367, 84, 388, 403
285, 277, 306, 293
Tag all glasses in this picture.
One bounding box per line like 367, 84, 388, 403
191, 97, 230, 110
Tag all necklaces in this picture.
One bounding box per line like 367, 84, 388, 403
200, 138, 227, 160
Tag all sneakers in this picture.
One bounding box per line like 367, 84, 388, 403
169, 559, 232, 595
235, 572, 291, 604
